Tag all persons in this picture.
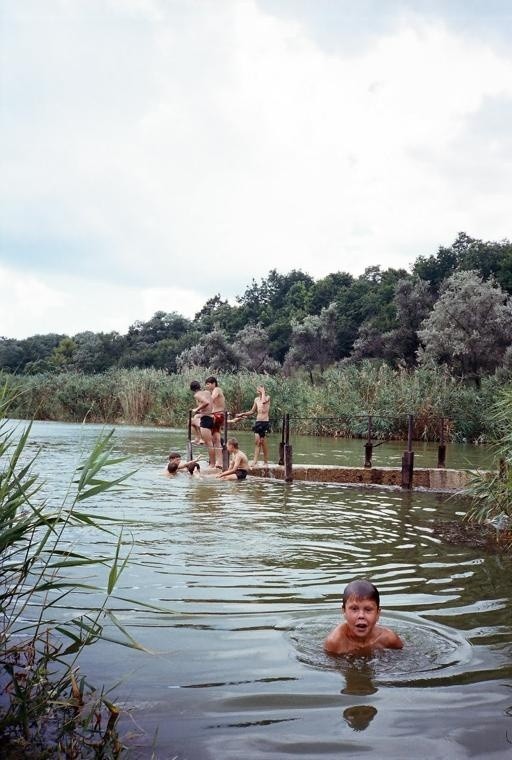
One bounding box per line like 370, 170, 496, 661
214, 436, 250, 481
166, 451, 201, 470
194, 376, 226, 469
187, 464, 202, 477
159, 462, 179, 478
189, 380, 216, 470
235, 384, 272, 468
321, 577, 404, 662
336, 660, 380, 733
